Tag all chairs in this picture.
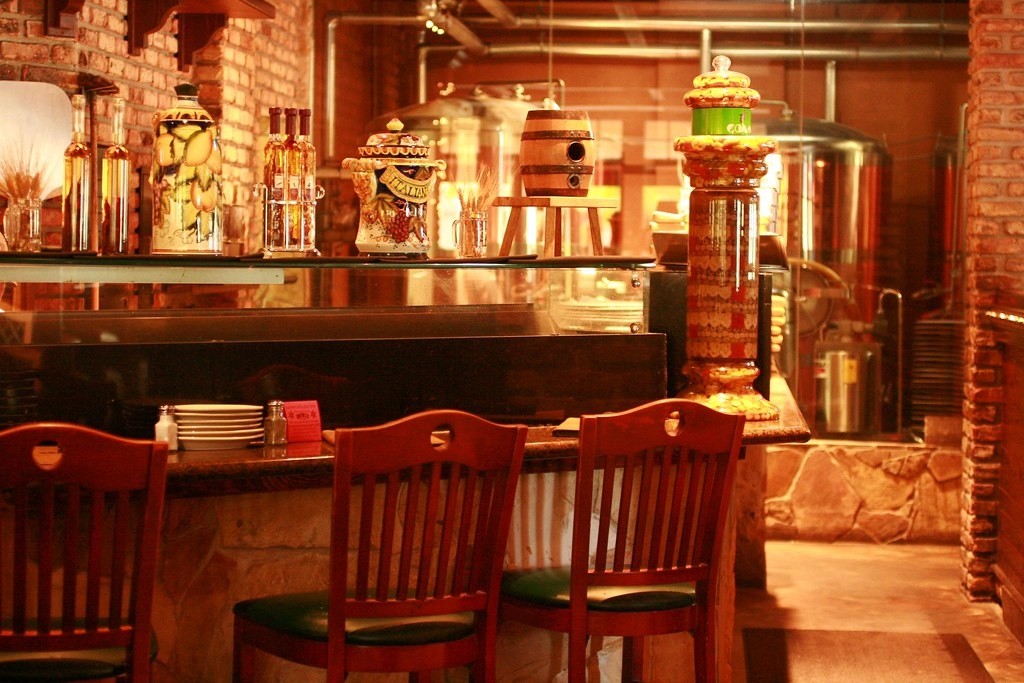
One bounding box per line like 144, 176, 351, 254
496, 399, 746, 683
0, 422, 168, 683
233, 409, 528, 683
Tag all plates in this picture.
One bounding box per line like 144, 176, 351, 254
175, 405, 264, 450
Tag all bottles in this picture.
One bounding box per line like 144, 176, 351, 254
154, 404, 178, 450
284, 107, 299, 251
298, 108, 316, 251
264, 107, 285, 251
100, 99, 127, 255
61, 93, 90, 251
265, 401, 286, 445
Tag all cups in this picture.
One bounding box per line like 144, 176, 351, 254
452, 210, 488, 259
3, 198, 41, 253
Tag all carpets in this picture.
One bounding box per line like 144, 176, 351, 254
741, 627, 995, 683
